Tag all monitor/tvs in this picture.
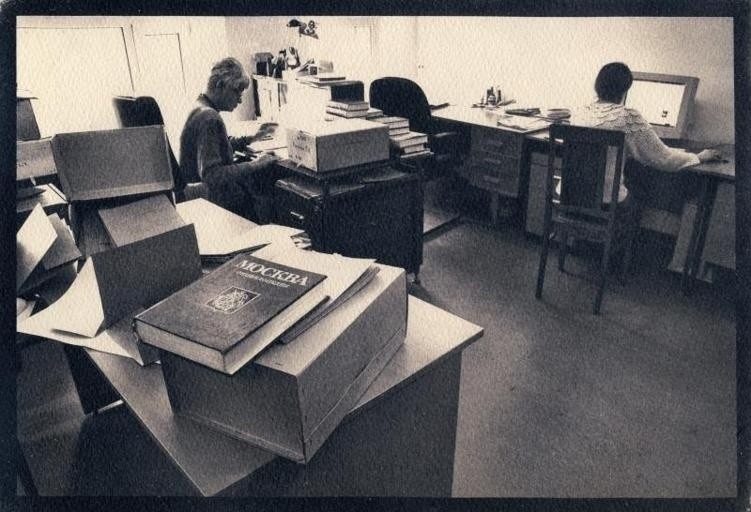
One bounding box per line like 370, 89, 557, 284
623, 70, 700, 141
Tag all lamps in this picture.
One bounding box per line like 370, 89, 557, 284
286, 18, 316, 39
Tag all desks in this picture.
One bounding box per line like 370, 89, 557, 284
218, 143, 427, 287
524, 121, 736, 296
37, 199, 484, 497
426, 102, 563, 220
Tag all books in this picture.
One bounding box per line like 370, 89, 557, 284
129, 250, 333, 375
246, 237, 380, 346
325, 95, 435, 163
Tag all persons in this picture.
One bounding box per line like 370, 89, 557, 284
556, 60, 728, 271
178, 54, 283, 226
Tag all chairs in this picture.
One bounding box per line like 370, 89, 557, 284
110, 91, 210, 202
533, 124, 642, 315
368, 75, 470, 234
